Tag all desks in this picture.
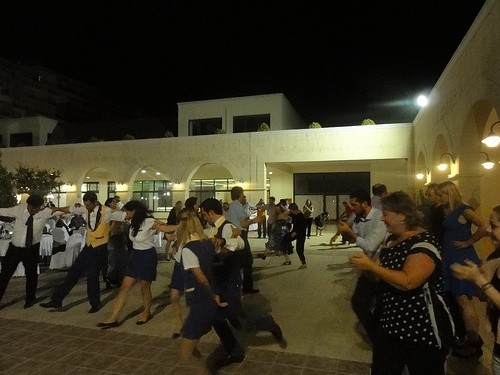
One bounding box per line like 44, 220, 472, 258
0, 239, 40, 276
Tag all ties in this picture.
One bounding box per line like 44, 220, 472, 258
25, 214, 33, 248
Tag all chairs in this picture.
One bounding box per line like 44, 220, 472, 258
0, 211, 161, 278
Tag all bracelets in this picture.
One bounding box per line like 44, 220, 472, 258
472, 234, 478, 242
481, 282, 492, 293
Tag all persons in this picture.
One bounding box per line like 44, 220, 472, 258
330, 181, 500, 375
0, 187, 329, 363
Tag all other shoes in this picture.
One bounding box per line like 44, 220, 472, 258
263, 315, 283, 340
452, 335, 484, 359
165, 256, 171, 261
39, 300, 62, 308
89, 302, 103, 313
136, 312, 151, 325
241, 287, 260, 293
24, 300, 34, 308
97, 321, 117, 326
217, 352, 243, 367
283, 261, 291, 266
298, 263, 307, 270
257, 253, 267, 260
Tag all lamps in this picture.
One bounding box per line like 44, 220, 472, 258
480, 151, 495, 170
436, 152, 455, 171
481, 121, 500, 148
416, 167, 429, 179
